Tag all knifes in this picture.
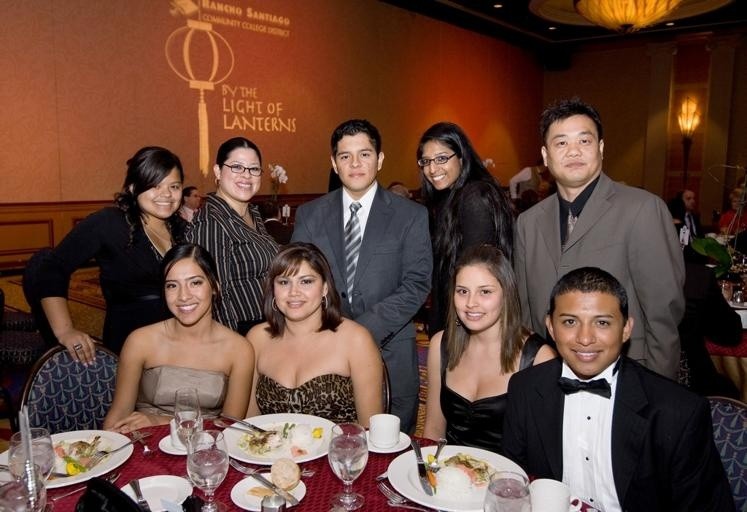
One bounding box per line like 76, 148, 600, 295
411, 440, 433, 496
251, 473, 300, 506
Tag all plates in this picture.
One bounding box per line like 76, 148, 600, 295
120, 474, 195, 512
0, 430, 135, 490
158, 431, 215, 456
363, 430, 412, 454
385, 445, 531, 512
215, 413, 343, 466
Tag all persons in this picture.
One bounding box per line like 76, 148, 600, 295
719, 189, 747, 240
419, 243, 557, 454
670, 188, 701, 244
242, 243, 387, 429
103, 243, 253, 434
39, 146, 193, 370
513, 101, 689, 380
508, 154, 551, 206
288, 118, 434, 436
183, 137, 282, 336
414, 122, 518, 344
387, 178, 413, 202
176, 185, 201, 223
506, 267, 737, 512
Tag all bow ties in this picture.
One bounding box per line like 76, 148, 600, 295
558, 376, 611, 399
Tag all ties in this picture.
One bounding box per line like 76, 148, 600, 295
344, 203, 361, 300
563, 209, 576, 246
687, 214, 695, 236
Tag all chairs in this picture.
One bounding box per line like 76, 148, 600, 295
707, 397, 747, 512
382, 357, 389, 416
15, 341, 121, 445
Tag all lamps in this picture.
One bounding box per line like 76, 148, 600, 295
575, 0, 680, 32
678, 95, 701, 184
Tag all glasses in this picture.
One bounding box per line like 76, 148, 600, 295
417, 152, 459, 167
219, 163, 264, 176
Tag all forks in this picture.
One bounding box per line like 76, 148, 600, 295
228, 458, 315, 477
376, 481, 411, 504
427, 439, 447, 472
87, 431, 154, 470
132, 429, 154, 461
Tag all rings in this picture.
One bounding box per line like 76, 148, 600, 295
73, 343, 84, 351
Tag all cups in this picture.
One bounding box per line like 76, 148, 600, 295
7, 429, 55, 482
368, 413, 401, 450
528, 478, 583, 512
259, 495, 288, 512
186, 430, 231, 512
1, 461, 48, 511
169, 418, 188, 451
327, 421, 370, 511
175, 387, 203, 488
483, 471, 528, 512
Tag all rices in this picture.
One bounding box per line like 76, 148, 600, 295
288, 423, 315, 453
435, 468, 472, 497
